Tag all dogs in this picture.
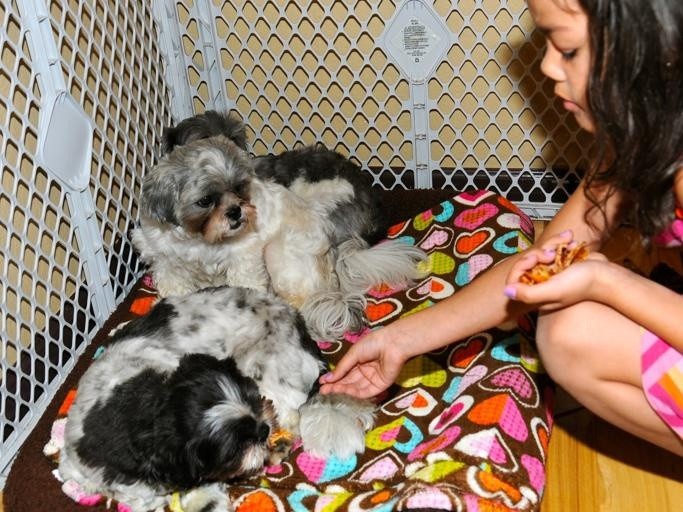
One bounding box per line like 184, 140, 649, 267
131, 110, 428, 342
59, 285, 379, 512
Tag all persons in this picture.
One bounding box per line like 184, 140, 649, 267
316, 2, 683, 459
